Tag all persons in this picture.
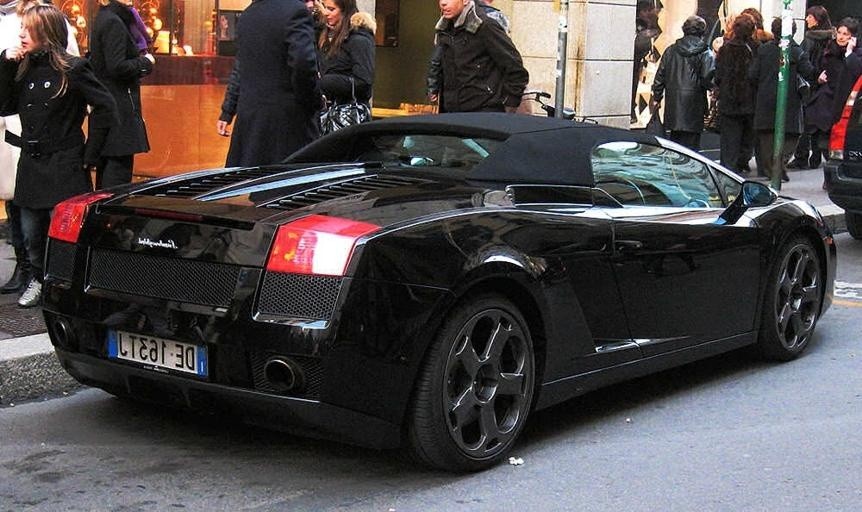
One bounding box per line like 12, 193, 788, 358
425, 0, 530, 115
0, 1, 156, 308
630, 0, 861, 184
216, 0, 377, 168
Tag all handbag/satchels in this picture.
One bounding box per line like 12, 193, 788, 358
645, 104, 667, 140
795, 71, 812, 95
318, 75, 373, 139
703, 88, 723, 136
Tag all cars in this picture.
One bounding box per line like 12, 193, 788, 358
821, 74, 861, 241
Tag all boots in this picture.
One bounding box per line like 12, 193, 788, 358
0, 200, 29, 295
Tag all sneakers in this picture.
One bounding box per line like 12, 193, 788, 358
785, 157, 822, 170
16, 276, 43, 309
764, 169, 790, 183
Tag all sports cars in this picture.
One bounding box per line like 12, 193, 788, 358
40, 109, 837, 477
